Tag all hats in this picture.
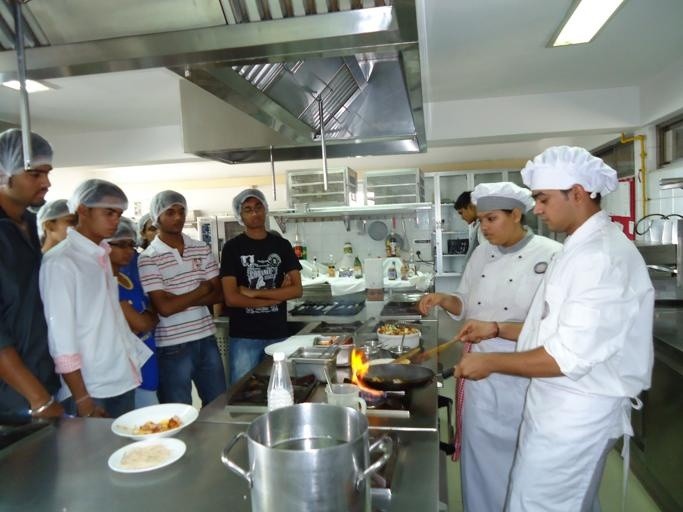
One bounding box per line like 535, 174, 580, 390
0, 129, 53, 185
140, 213, 151, 231
150, 190, 186, 228
470, 181, 536, 215
105, 222, 135, 241
520, 146, 618, 198
37, 199, 70, 224
66, 179, 128, 214
232, 189, 268, 227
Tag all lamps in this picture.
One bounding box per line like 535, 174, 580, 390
545, 0, 626, 50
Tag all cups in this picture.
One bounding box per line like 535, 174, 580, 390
324, 384, 366, 416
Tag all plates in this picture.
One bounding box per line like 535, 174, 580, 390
108, 438, 186, 473
111, 404, 199, 440
313, 335, 354, 349
285, 347, 339, 364
226, 374, 316, 413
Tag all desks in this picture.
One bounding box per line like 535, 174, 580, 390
212, 300, 438, 391
2, 417, 440, 512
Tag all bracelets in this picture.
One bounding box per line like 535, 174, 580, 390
28, 397, 55, 413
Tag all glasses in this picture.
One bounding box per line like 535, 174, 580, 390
243, 204, 264, 212
108, 240, 137, 248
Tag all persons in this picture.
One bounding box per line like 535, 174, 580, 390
221, 187, 302, 384
138, 191, 227, 405
453, 190, 488, 245
457, 143, 654, 511
38, 179, 142, 416
137, 214, 156, 249
102, 222, 157, 410
0, 128, 70, 429
423, 182, 562, 511
37, 199, 74, 254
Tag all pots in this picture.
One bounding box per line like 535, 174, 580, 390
356, 364, 457, 392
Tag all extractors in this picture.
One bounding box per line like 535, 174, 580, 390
179, 46, 421, 164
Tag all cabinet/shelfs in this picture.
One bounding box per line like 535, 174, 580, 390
198, 216, 244, 268
269, 202, 436, 301
615, 308, 683, 512
423, 167, 557, 278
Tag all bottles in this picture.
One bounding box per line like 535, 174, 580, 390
267, 352, 294, 412
390, 232, 396, 256
302, 242, 307, 259
408, 249, 415, 279
294, 232, 302, 260
303, 203, 310, 214
387, 261, 395, 279
312, 256, 319, 279
328, 254, 335, 277
400, 259, 407, 280
353, 254, 362, 279
